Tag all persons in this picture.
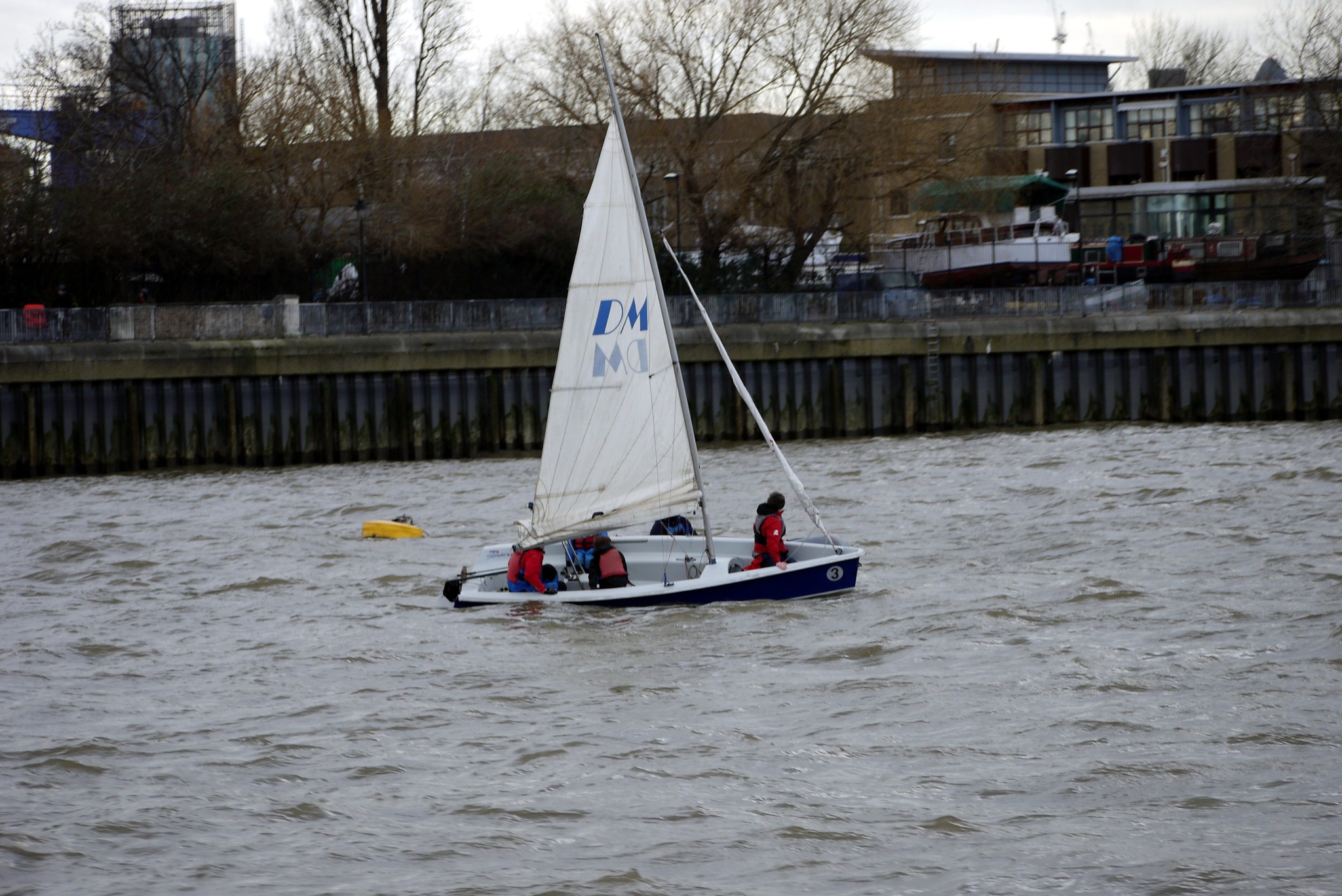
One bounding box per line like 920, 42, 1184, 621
507, 543, 567, 594
588, 535, 628, 590
650, 515, 698, 535
751, 491, 797, 570
51, 285, 76, 341
567, 532, 607, 573
353, 291, 366, 334
314, 289, 324, 302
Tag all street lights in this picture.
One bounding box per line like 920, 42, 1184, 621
660, 172, 685, 293
344, 199, 370, 336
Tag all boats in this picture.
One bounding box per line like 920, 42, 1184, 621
1050, 175, 1328, 282
885, 172, 1080, 289
667, 225, 885, 290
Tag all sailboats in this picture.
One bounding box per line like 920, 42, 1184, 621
441, 32, 864, 609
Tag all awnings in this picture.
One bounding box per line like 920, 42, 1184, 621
1117, 100, 1176, 111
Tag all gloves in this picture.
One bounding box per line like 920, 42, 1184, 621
545, 588, 557, 594
